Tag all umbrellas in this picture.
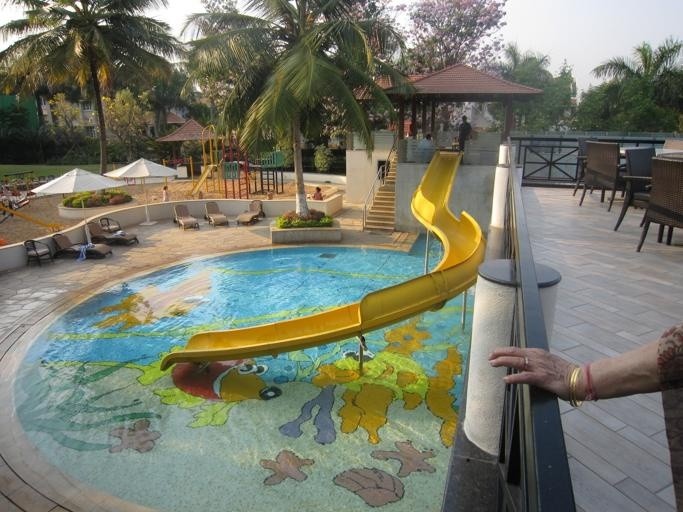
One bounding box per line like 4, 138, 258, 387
103, 157, 180, 221
30, 168, 128, 243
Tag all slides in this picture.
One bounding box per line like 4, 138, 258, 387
161, 149, 484, 372
190, 166, 214, 194
0, 204, 63, 230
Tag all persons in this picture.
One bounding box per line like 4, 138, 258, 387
458, 115, 472, 151
312, 186, 323, 200
486, 324, 683, 512
421, 134, 434, 147
162, 186, 170, 202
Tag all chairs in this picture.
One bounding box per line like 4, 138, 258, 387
173, 200, 263, 230
22, 216, 138, 267
574, 138, 681, 253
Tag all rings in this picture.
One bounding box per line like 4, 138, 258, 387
525, 356, 529, 370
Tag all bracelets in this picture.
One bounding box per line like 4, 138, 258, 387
569, 360, 596, 408
